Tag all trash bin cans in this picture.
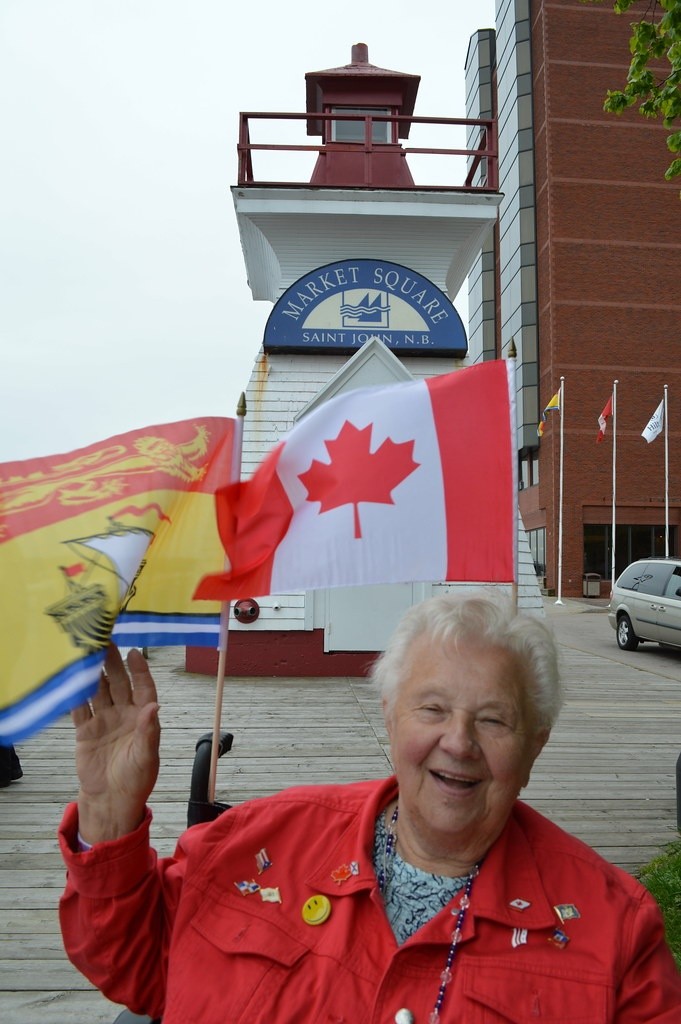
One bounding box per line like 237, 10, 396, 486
583, 573, 601, 598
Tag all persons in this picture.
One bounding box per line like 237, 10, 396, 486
56, 590, 681, 1024
0, 747, 23, 788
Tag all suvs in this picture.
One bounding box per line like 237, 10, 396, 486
607, 556, 681, 653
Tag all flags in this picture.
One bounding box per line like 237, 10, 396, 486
195, 359, 521, 601
537, 381, 564, 437
641, 397, 667, 444
596, 384, 616, 445
0, 416, 242, 747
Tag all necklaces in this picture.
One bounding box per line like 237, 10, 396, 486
378, 806, 479, 1023
